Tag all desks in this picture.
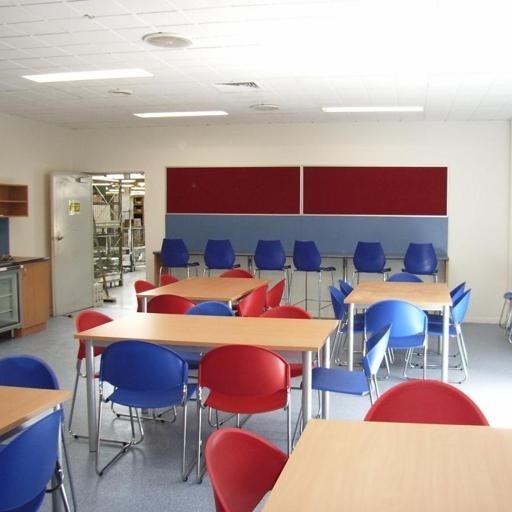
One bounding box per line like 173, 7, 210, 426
136, 277, 271, 313
74, 319, 340, 452
343, 280, 453, 384
262, 419, 512, 512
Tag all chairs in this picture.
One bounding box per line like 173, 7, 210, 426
499, 291, 512, 344
0, 385, 73, 445
364, 379, 491, 426
328, 279, 395, 381
0, 354, 78, 512
67, 308, 178, 443
95, 339, 200, 481
151, 302, 325, 450
292, 322, 393, 451
195, 345, 302, 483
0, 408, 71, 512
360, 300, 428, 380
134, 238, 439, 318
402, 279, 472, 383
204, 427, 290, 512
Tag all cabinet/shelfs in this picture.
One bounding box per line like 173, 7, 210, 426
0, 255, 52, 342
0, 184, 29, 218
92, 179, 124, 288
